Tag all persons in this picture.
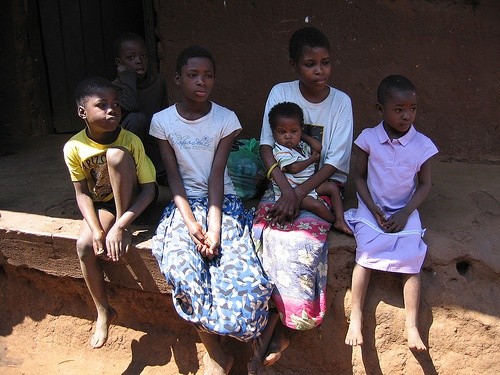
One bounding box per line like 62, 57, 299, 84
63, 77, 156, 349
268, 102, 353, 236
247, 28, 353, 374
110, 39, 165, 184
148, 46, 273, 375
345, 75, 439, 349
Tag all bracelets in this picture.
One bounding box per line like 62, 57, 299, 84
266, 162, 278, 179
